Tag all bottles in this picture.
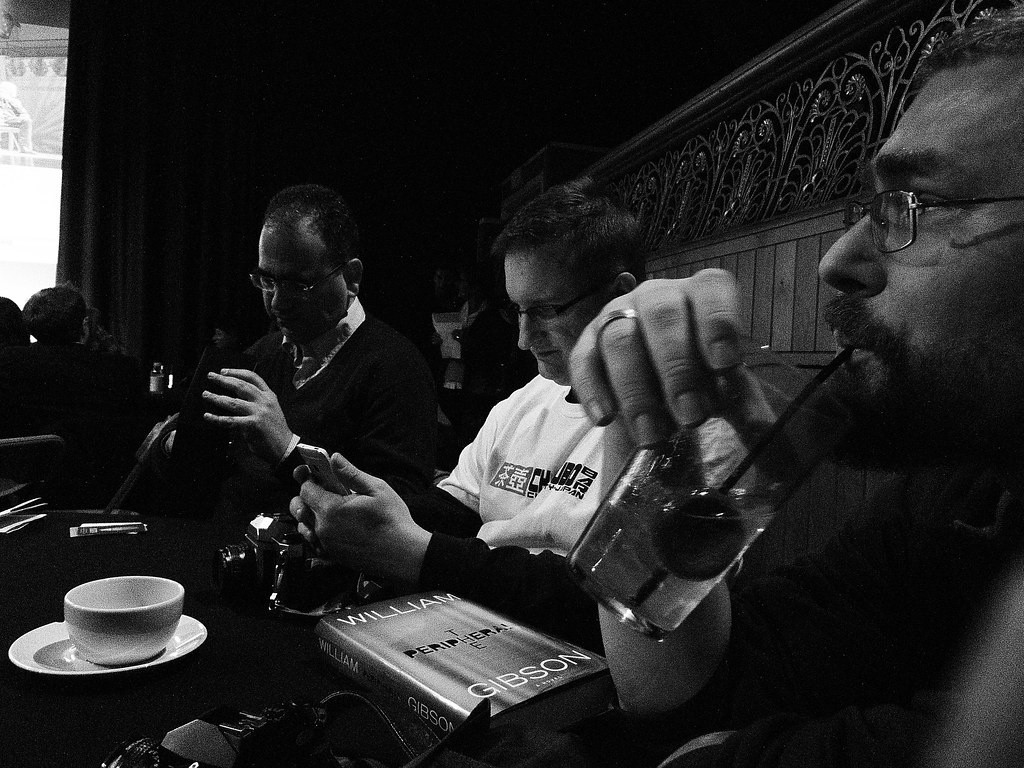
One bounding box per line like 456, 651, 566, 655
149, 362, 165, 397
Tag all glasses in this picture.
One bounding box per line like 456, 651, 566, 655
843, 190, 1024, 253
498, 285, 608, 324
250, 263, 342, 296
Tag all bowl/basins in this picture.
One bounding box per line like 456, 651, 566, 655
64, 576, 185, 665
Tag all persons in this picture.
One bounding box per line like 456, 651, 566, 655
1, 178, 658, 636
568, 17, 1024, 768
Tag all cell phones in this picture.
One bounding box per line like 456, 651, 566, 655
297, 443, 352, 495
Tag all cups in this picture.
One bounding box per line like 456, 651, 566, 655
565, 333, 842, 637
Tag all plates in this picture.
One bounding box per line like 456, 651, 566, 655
8, 614, 208, 676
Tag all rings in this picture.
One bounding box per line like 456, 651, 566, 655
595, 308, 637, 335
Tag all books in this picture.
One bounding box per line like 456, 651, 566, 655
314, 589, 618, 738
